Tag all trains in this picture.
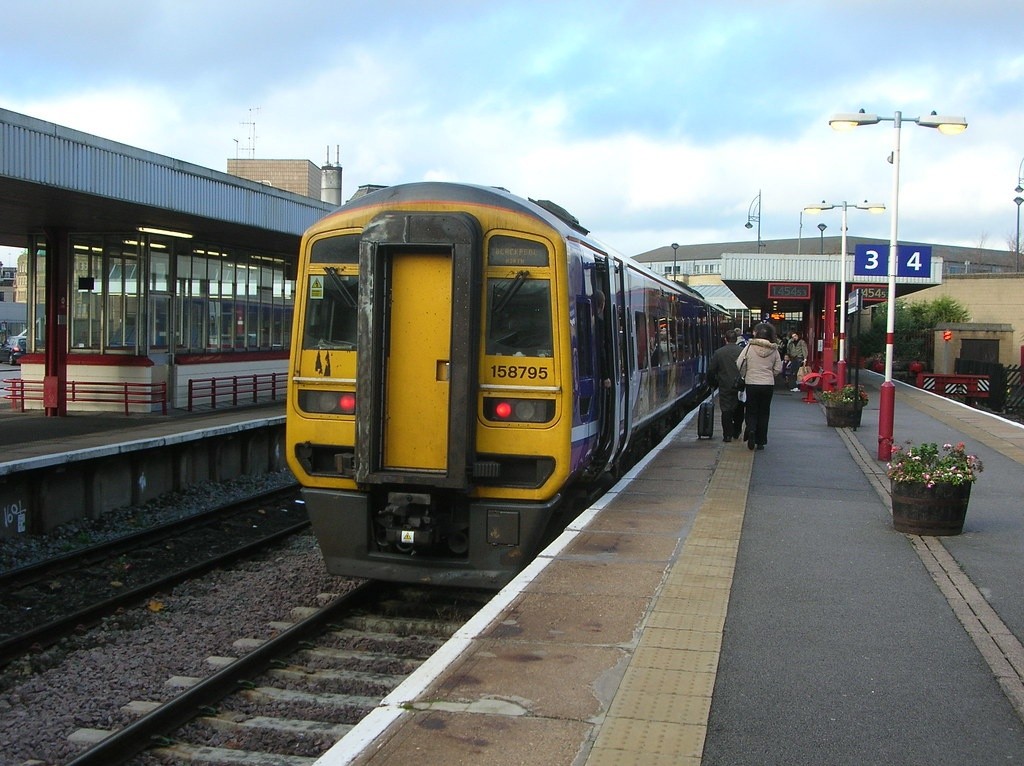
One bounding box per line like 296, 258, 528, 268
277, 181, 740, 594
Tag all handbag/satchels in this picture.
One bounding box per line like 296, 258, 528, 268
735, 375, 746, 393
797, 361, 812, 382
738, 388, 746, 402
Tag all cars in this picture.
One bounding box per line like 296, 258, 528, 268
0, 336, 41, 366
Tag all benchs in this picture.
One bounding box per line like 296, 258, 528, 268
795, 366, 824, 403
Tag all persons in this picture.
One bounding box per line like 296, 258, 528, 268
780, 332, 808, 392
707, 330, 744, 442
736, 322, 782, 449
650, 327, 677, 404
734, 327, 749, 347
594, 289, 611, 388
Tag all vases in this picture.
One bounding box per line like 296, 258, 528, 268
889, 480, 972, 536
824, 406, 863, 428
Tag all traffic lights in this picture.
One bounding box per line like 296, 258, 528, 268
943, 331, 950, 342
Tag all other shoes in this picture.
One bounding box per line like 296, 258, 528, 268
731, 424, 741, 439
748, 431, 755, 450
723, 435, 732, 442
790, 387, 801, 392
756, 444, 764, 451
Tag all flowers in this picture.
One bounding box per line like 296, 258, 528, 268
818, 384, 870, 408
885, 438, 987, 490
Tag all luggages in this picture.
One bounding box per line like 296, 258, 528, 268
698, 387, 715, 439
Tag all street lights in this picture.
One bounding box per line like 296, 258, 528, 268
671, 242, 680, 286
1014, 196, 1024, 270
804, 199, 886, 397
817, 222, 827, 255
825, 109, 969, 465
744, 189, 767, 258
